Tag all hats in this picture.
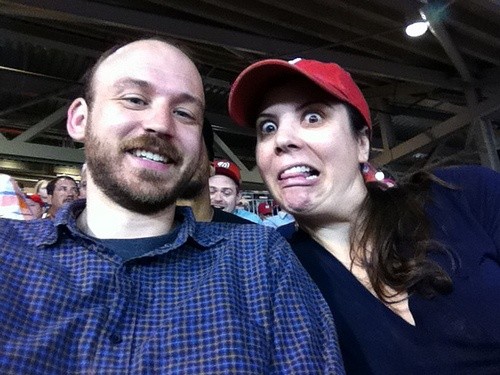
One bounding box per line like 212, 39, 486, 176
229, 57, 373, 133
213, 159, 241, 187
258, 202, 272, 214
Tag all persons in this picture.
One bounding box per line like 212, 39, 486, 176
176, 116, 256, 224
1, 161, 85, 220
0, 33, 347, 375
258, 202, 274, 220
206, 158, 262, 226
263, 205, 295, 229
227, 58, 500, 375
